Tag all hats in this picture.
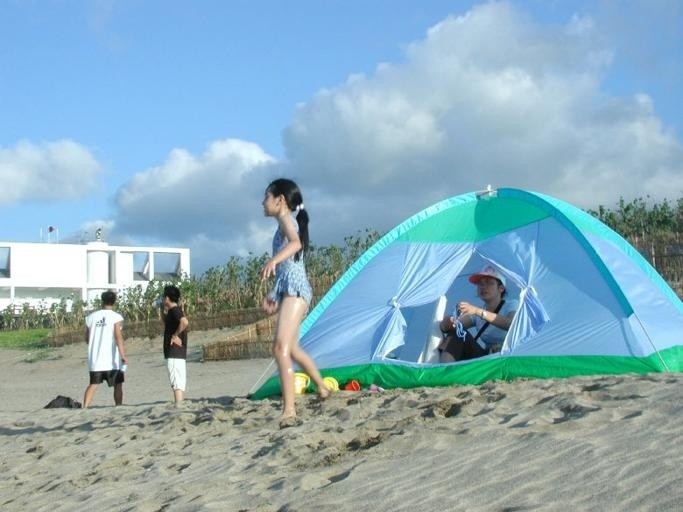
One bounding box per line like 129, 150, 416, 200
469, 264, 506, 287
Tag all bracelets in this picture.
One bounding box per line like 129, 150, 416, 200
480, 309, 487, 320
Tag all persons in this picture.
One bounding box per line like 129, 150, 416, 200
81, 290, 127, 408
436, 263, 520, 364
158, 284, 189, 410
255, 177, 331, 427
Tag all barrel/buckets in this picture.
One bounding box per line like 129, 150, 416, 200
294, 373, 309, 396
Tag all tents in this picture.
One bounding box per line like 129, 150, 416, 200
246, 187, 683, 399
